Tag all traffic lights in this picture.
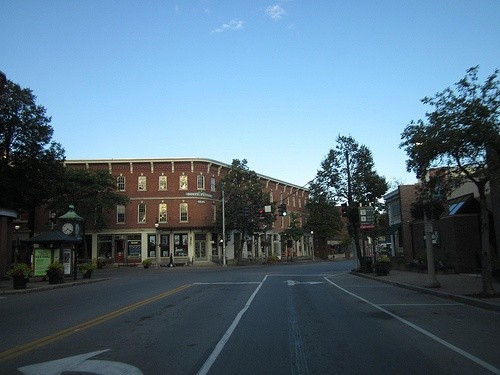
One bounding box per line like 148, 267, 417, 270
278, 203, 286, 217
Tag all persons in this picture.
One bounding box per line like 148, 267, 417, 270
168, 253, 175, 267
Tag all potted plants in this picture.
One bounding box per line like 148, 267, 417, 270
79, 263, 95, 279
142, 260, 151, 269
45, 261, 64, 284
5, 263, 32, 289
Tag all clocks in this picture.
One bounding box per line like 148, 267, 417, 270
75, 223, 80, 235
62, 223, 73, 235
